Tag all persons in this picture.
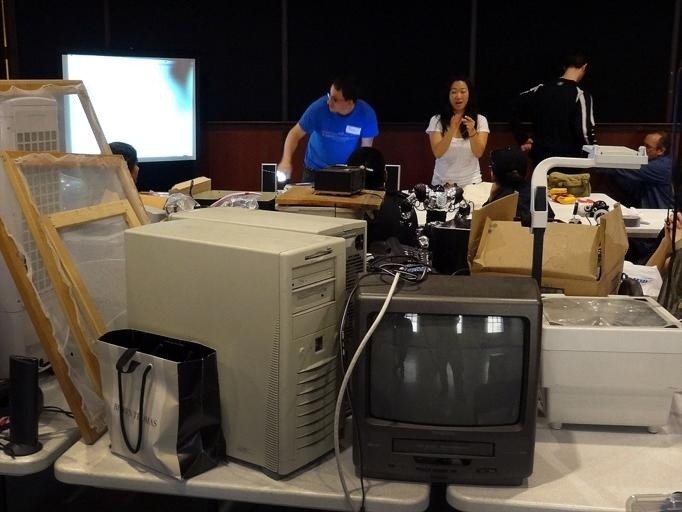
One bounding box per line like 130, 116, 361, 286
644, 208, 682, 323
81, 141, 142, 208
387, 313, 412, 378
276, 75, 378, 185
607, 124, 675, 210
425, 74, 496, 194
419, 314, 468, 405
507, 47, 600, 165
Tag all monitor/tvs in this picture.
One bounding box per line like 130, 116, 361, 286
355, 271, 544, 487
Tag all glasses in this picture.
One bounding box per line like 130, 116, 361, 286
327, 90, 350, 104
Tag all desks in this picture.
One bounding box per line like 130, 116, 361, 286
619, 207, 671, 241
0, 369, 81, 512
57, 427, 430, 512
445, 408, 681, 512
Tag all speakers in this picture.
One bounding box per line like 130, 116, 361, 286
382, 163, 400, 191
260, 162, 278, 191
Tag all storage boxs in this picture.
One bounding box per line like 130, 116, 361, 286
467, 189, 624, 296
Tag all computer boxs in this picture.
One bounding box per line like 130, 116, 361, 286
124, 220, 347, 479
173, 206, 369, 416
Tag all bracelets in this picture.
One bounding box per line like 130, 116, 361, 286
468, 131, 479, 139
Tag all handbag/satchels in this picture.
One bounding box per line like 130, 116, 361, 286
97, 328, 228, 482
